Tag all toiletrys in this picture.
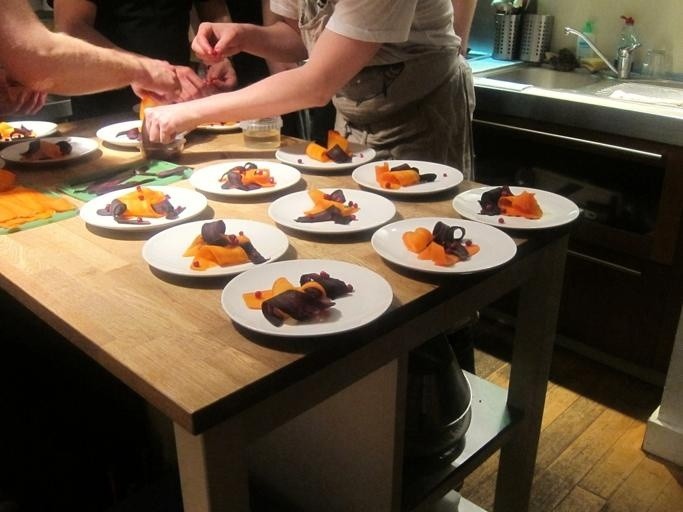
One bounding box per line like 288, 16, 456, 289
574, 20, 596, 61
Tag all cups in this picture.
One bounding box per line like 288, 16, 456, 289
243, 116, 281, 149
639, 49, 665, 79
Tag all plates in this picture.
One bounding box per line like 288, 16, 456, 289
275, 140, 377, 171
143, 217, 290, 278
97, 120, 187, 146
453, 185, 580, 229
219, 260, 395, 339
1, 136, 100, 165
372, 216, 516, 274
0, 121, 58, 143
352, 160, 463, 194
133, 102, 259, 131
124, 186, 207, 232
267, 188, 394, 233
189, 159, 302, 197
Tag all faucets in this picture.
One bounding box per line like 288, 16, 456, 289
564, 24, 617, 77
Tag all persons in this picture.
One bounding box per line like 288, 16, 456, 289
0, 2, 182, 116
264, 1, 476, 143
141, 1, 476, 181
54, 0, 237, 118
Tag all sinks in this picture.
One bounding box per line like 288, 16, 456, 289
473, 62, 604, 90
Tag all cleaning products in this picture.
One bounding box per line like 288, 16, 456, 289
614, 16, 634, 68
